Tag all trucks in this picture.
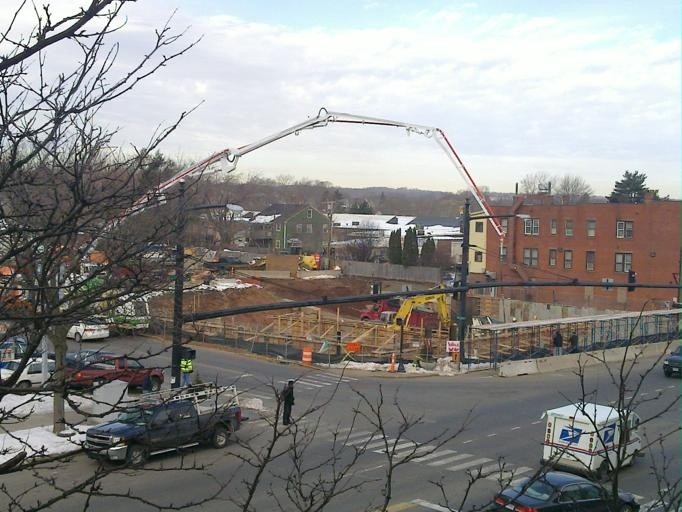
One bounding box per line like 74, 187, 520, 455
540, 402, 642, 479
361, 298, 438, 329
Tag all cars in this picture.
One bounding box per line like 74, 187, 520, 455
663, 346, 682, 378
491, 472, 640, 512
67, 316, 110, 342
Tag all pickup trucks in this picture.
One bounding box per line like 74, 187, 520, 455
82, 400, 241, 468
0, 342, 164, 394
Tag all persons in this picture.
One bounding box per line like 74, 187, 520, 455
552, 330, 563, 356
283, 381, 295, 426
568, 331, 578, 353
180, 359, 194, 388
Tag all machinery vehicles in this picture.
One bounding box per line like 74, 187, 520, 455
65, 275, 150, 335
388, 284, 499, 336
